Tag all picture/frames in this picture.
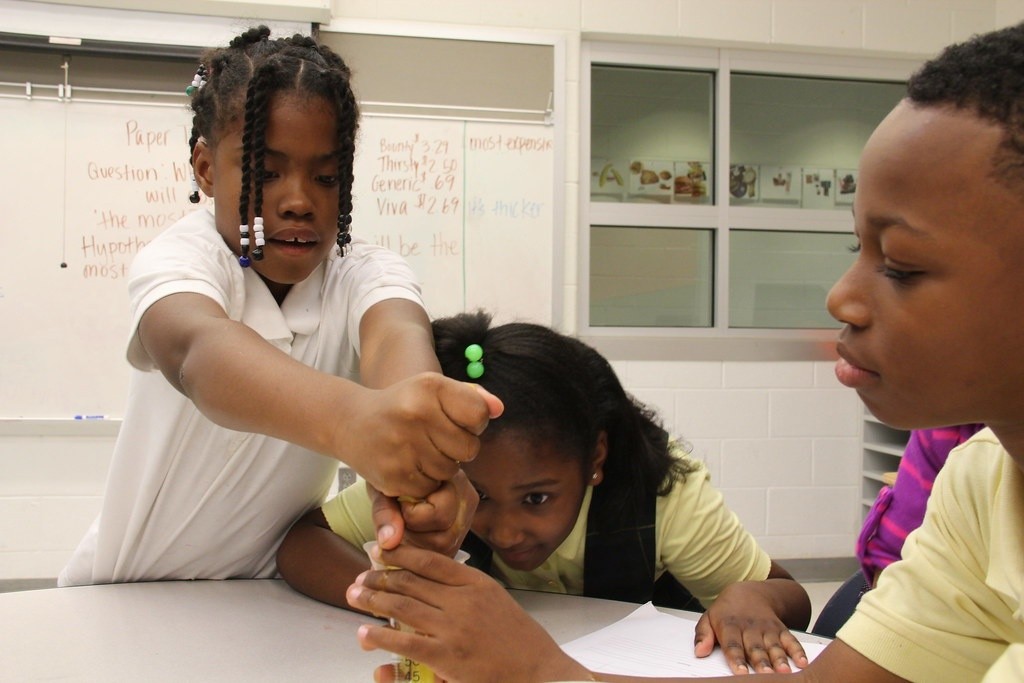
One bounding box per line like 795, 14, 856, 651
590, 155, 860, 214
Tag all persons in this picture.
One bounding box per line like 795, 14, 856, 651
856, 420, 987, 588
277, 309, 812, 678
57, 26, 502, 589
345, 19, 1024, 682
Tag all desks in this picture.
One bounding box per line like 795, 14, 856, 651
0, 584, 834, 683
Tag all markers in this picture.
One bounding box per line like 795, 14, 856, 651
74, 414, 109, 420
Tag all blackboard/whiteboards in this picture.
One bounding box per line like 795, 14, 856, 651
0, 79, 566, 441
